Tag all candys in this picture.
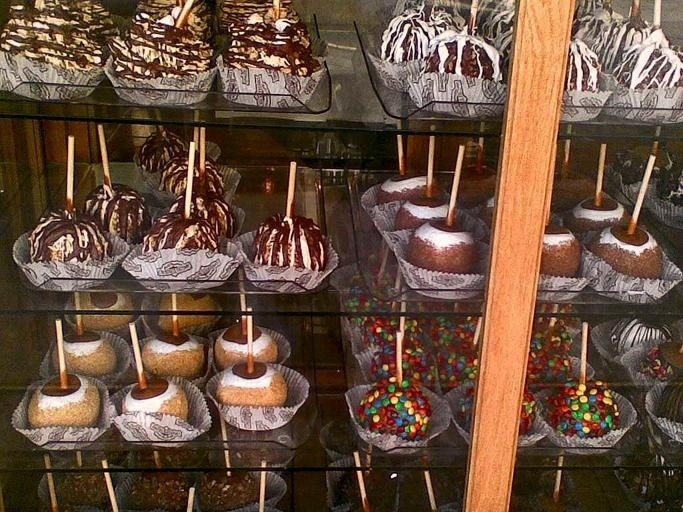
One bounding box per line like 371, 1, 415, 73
609, 316, 681, 425
345, 255, 622, 444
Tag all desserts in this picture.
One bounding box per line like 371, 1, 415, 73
29, 126, 239, 289
486, 164, 663, 279
0, 0, 318, 108
379, 1, 682, 124
29, 292, 288, 427
254, 214, 327, 273
612, 147, 682, 208
325, 418, 681, 511
377, 173, 481, 274
48, 433, 290, 510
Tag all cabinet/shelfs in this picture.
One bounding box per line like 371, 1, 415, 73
2, 0, 683, 512
